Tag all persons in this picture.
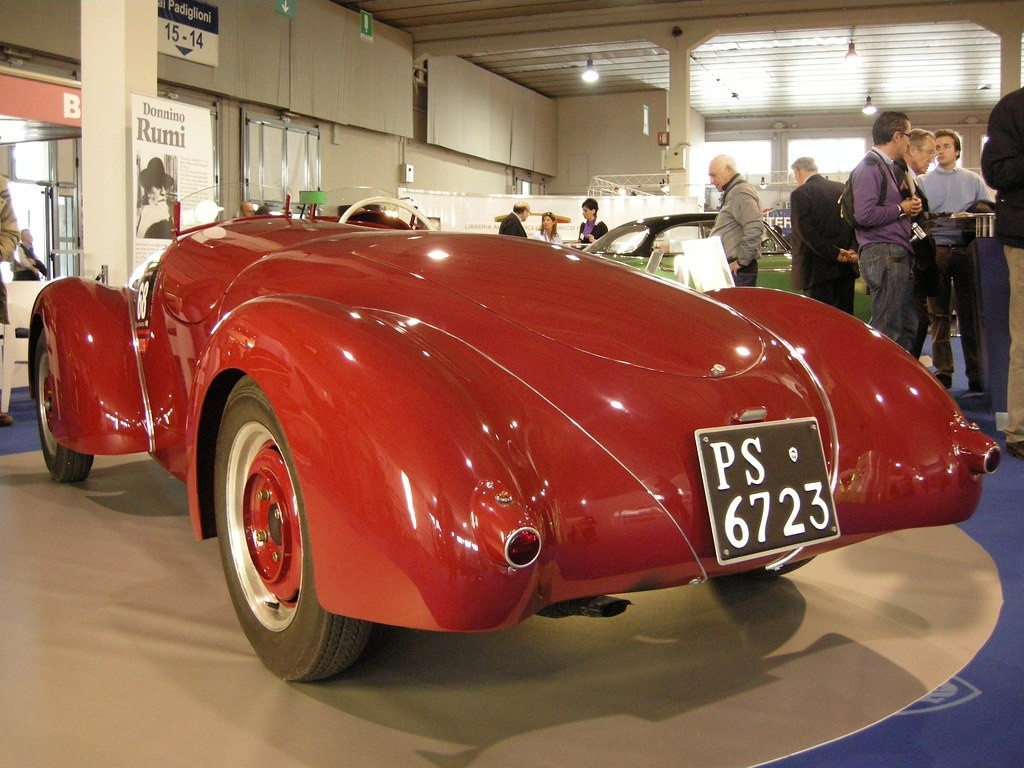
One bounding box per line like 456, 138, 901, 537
240, 201, 255, 217
790, 157, 860, 316
13, 229, 47, 281
848, 110, 951, 364
981, 88, 1024, 460
915, 129, 993, 399
707, 154, 764, 287
531, 211, 562, 249
498, 201, 530, 237
0, 173, 21, 425
577, 198, 608, 244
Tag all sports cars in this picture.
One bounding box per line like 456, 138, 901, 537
26, 181, 1002, 684
567, 211, 873, 328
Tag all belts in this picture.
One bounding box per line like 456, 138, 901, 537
936, 244, 965, 250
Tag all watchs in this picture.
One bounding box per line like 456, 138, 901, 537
896, 205, 907, 221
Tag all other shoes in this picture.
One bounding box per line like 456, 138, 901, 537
938, 376, 952, 386
1006, 442, 1024, 459
0, 414, 14, 425
969, 388, 982, 398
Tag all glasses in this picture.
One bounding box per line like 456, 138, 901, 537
920, 147, 937, 159
901, 133, 912, 140
526, 211, 531, 215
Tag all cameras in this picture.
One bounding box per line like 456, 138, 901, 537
911, 222, 926, 239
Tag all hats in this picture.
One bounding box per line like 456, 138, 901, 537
139, 157, 174, 186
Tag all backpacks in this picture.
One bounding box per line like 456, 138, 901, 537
838, 158, 886, 232
